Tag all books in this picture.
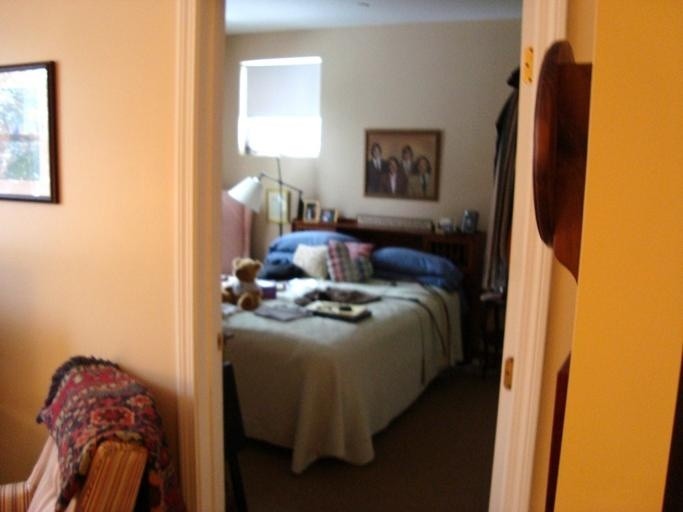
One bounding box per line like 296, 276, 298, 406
305, 299, 366, 317
311, 310, 373, 323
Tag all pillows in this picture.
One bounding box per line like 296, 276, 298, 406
264, 231, 464, 293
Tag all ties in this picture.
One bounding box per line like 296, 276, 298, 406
422, 174, 426, 193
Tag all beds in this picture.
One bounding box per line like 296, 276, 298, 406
218, 214, 488, 470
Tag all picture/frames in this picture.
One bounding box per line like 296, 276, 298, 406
0, 61, 59, 207
362, 129, 443, 202
301, 200, 340, 225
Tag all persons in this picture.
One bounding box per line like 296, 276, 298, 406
380, 155, 407, 196
368, 142, 389, 192
407, 154, 433, 196
400, 144, 419, 183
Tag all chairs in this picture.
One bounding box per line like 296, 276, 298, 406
3, 356, 160, 512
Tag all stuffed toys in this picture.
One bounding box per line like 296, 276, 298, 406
219, 255, 264, 311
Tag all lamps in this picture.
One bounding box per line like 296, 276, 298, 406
222, 173, 305, 221
